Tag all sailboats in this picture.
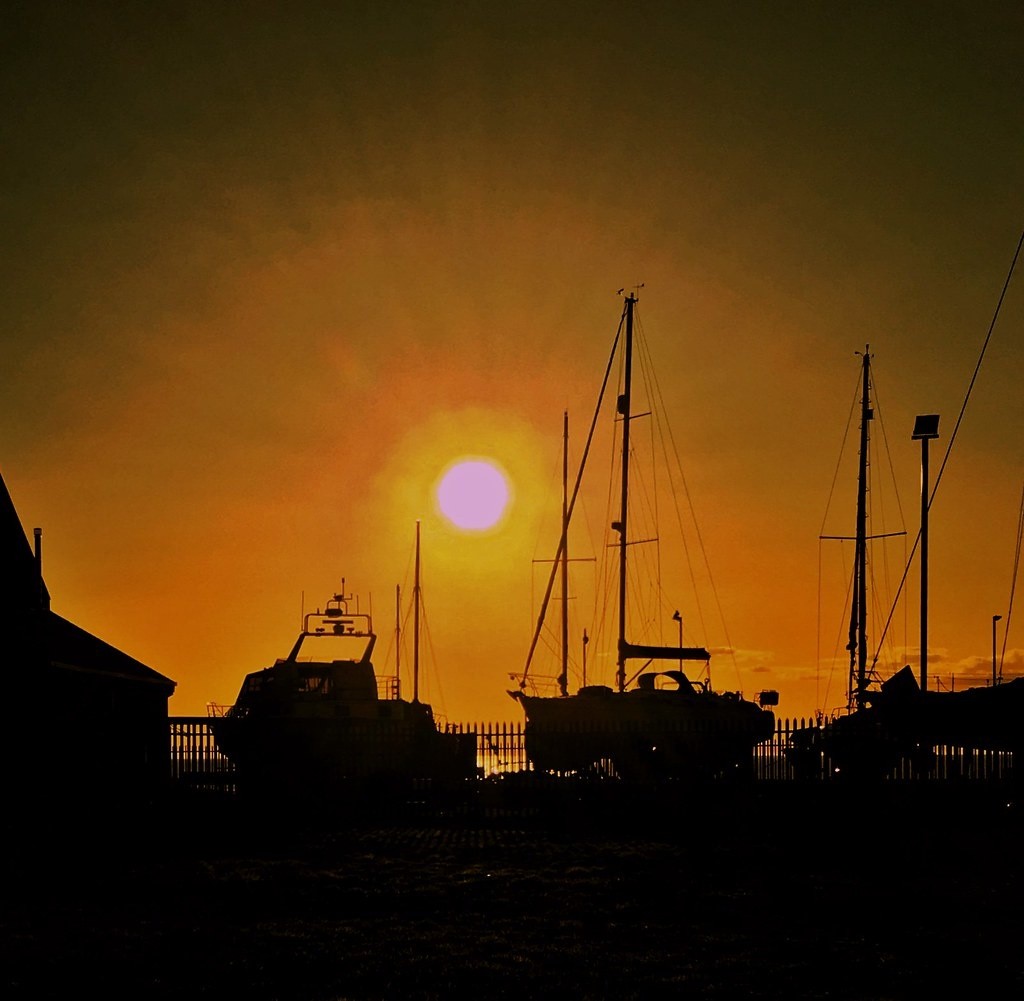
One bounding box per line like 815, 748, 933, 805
507, 284, 775, 780
206, 518, 477, 777
783, 342, 986, 782
